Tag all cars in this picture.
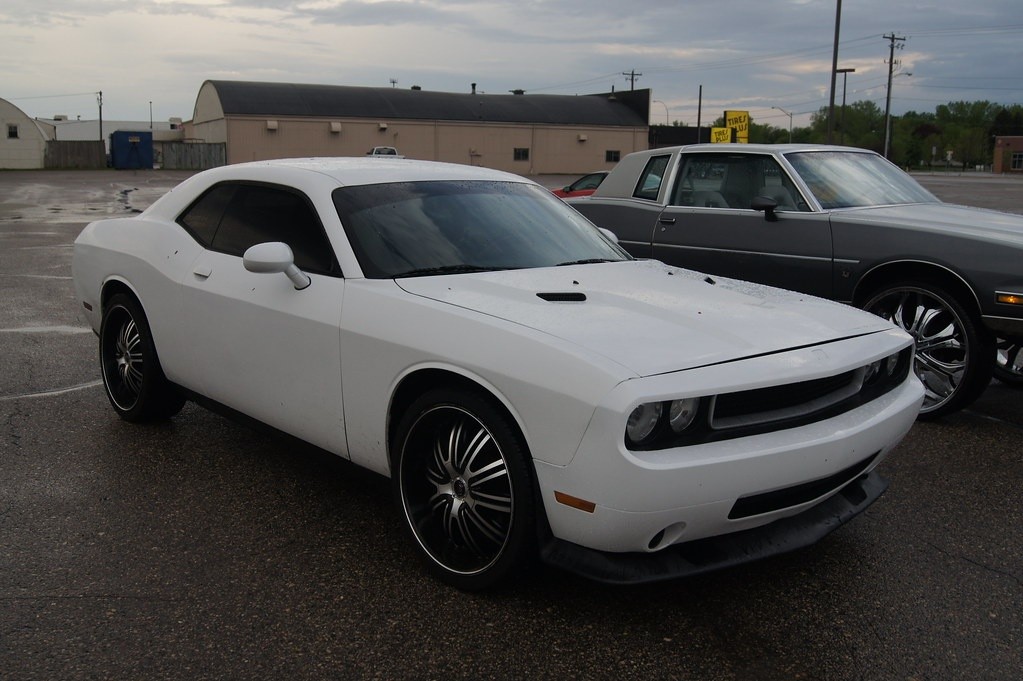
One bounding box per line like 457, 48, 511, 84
555, 139, 1023, 427
547, 170, 662, 198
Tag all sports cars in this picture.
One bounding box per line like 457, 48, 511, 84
69, 155, 928, 593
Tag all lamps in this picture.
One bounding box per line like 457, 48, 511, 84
379, 123, 388, 131
576, 134, 587, 142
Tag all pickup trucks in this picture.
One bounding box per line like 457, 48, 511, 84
364, 146, 405, 158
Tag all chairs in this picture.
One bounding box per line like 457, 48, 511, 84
758, 185, 800, 211
693, 190, 729, 208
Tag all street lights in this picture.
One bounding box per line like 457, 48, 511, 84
653, 100, 669, 126
837, 68, 855, 146
771, 106, 794, 143
883, 71, 913, 158
